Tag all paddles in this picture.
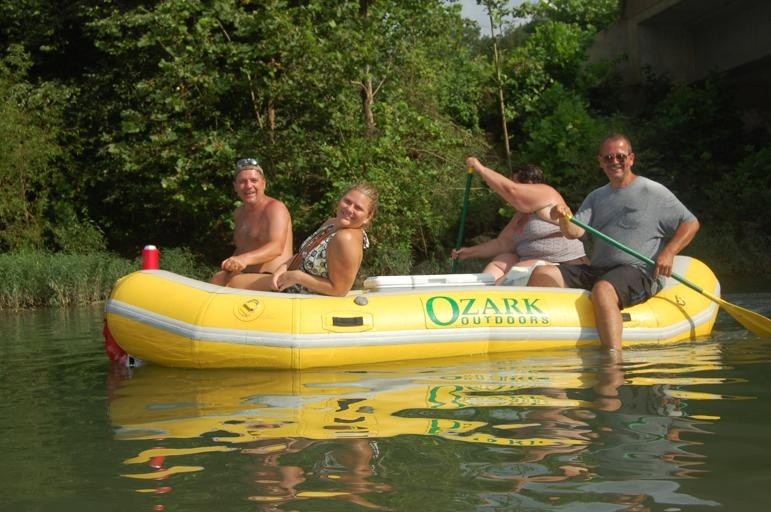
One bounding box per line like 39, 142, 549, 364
563, 211, 770, 338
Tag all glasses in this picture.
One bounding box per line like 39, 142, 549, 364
236, 158, 258, 168
600, 153, 630, 163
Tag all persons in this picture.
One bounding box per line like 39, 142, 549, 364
539, 130, 701, 365
473, 376, 592, 498
206, 154, 295, 294
228, 424, 312, 508
446, 155, 594, 289
264, 177, 382, 299
302, 432, 391, 512
581, 386, 699, 508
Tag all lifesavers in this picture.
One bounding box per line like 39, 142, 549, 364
102, 318, 149, 367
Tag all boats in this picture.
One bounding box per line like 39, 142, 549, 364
100, 249, 726, 376
96, 336, 726, 452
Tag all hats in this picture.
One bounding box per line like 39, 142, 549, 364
233, 164, 263, 176
